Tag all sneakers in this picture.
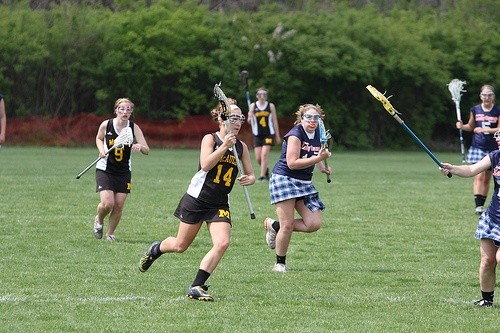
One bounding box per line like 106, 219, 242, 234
475, 206, 484, 214
263, 217, 277, 250
138, 241, 160, 272
273, 263, 287, 272
185, 285, 214, 302
477, 300, 493, 307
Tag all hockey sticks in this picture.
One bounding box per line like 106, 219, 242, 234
75, 126, 134, 180
318, 117, 332, 183
365, 84, 453, 179
240, 70, 258, 137
448, 79, 466, 163
214, 83, 257, 220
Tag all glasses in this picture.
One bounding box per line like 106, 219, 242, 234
116, 107, 133, 113
228, 114, 244, 125
480, 93, 494, 98
302, 114, 320, 122
493, 130, 500, 139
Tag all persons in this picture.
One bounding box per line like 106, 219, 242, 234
0, 96, 6, 145
247, 88, 281, 179
138, 98, 256, 301
456, 84, 500, 216
264, 103, 332, 271
438, 131, 500, 307
94, 98, 149, 240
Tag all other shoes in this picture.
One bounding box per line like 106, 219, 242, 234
257, 167, 269, 182
106, 234, 115, 239
94, 215, 103, 239
